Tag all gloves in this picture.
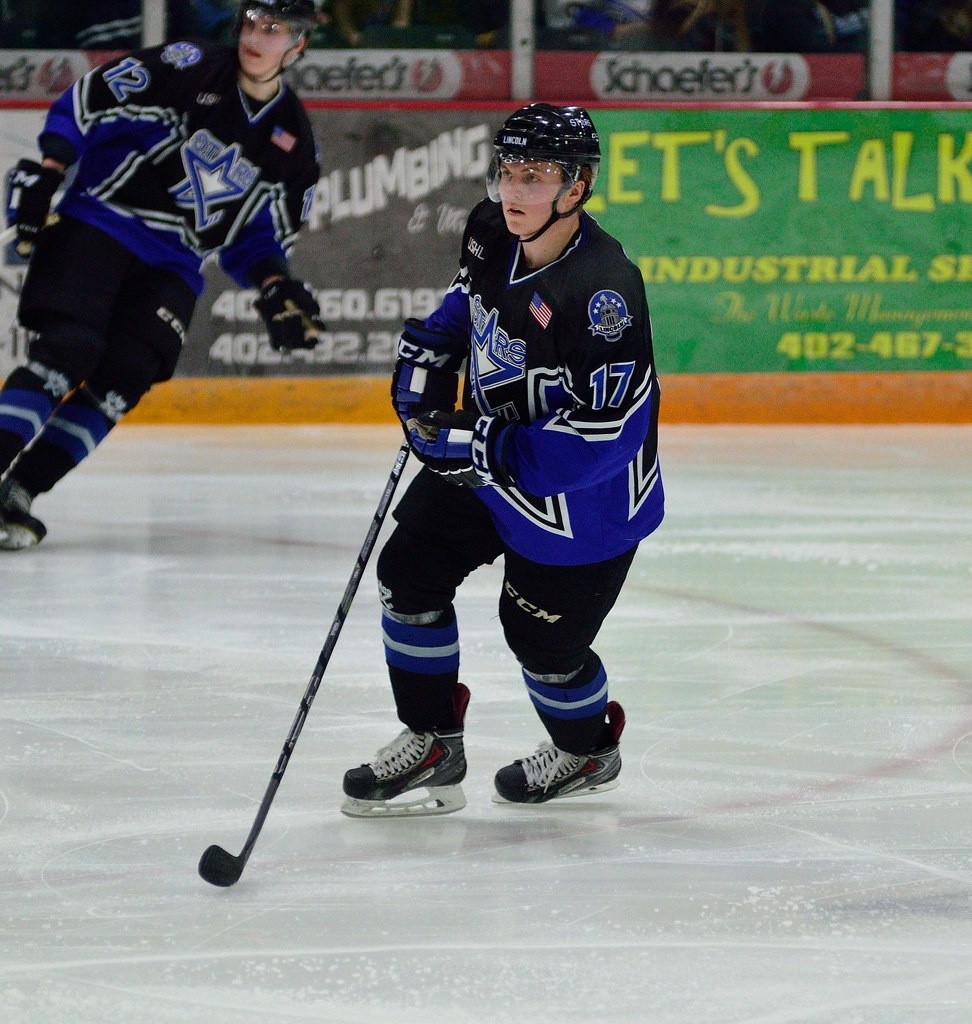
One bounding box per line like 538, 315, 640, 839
391, 316, 517, 490
5, 156, 64, 261
251, 278, 327, 356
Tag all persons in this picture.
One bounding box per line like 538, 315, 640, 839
343, 102, 665, 815
0, 0, 324, 552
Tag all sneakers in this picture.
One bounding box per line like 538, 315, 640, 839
340, 683, 472, 818
0, 468, 48, 551
491, 701, 627, 805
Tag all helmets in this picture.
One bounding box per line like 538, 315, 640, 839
492, 101, 602, 194
234, 0, 319, 69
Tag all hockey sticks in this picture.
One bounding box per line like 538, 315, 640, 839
199, 432, 411, 886
